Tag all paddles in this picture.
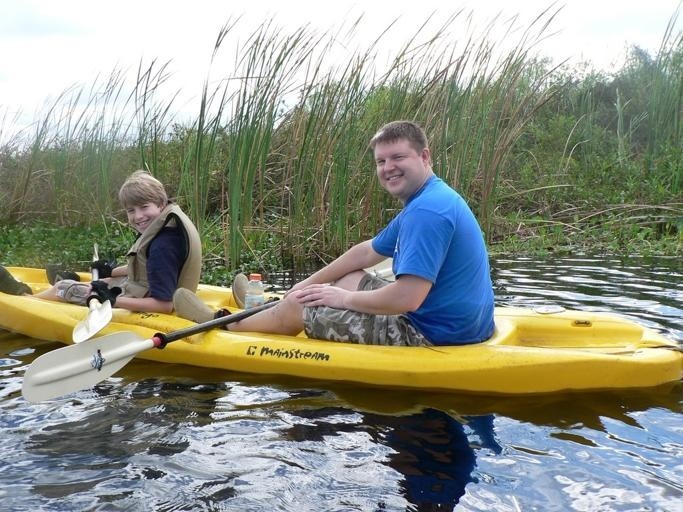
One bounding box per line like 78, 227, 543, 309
73, 243, 112, 344
21, 255, 395, 402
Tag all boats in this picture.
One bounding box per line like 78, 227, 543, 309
0, 267, 683, 397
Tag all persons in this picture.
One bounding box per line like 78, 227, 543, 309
0, 168, 201, 316
170, 119, 495, 346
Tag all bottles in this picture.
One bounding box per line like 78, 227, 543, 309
245, 273, 265, 310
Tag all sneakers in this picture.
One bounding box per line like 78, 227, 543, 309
0, 266, 31, 295
232, 272, 279, 308
45, 263, 67, 285
173, 287, 232, 330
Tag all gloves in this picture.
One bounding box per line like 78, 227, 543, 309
91, 259, 113, 278
92, 281, 116, 307
